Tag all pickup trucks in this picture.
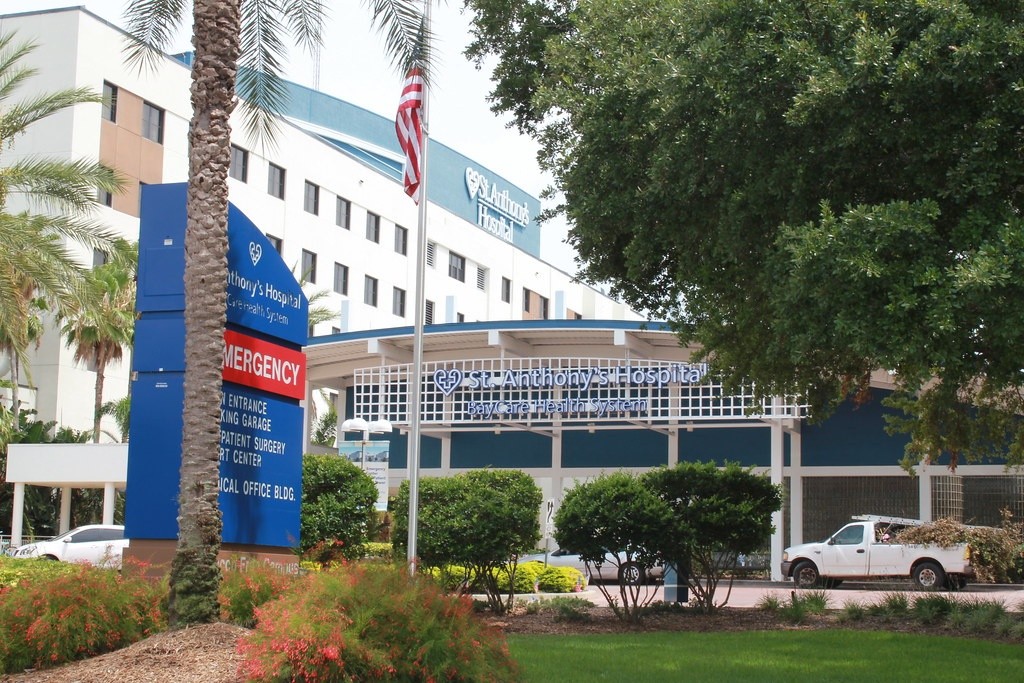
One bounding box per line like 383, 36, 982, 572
780, 523, 975, 591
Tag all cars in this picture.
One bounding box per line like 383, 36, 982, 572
12, 525, 130, 570
517, 547, 668, 585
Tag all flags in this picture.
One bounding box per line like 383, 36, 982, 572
394, 15, 423, 206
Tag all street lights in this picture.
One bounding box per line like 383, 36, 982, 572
340, 418, 393, 471
